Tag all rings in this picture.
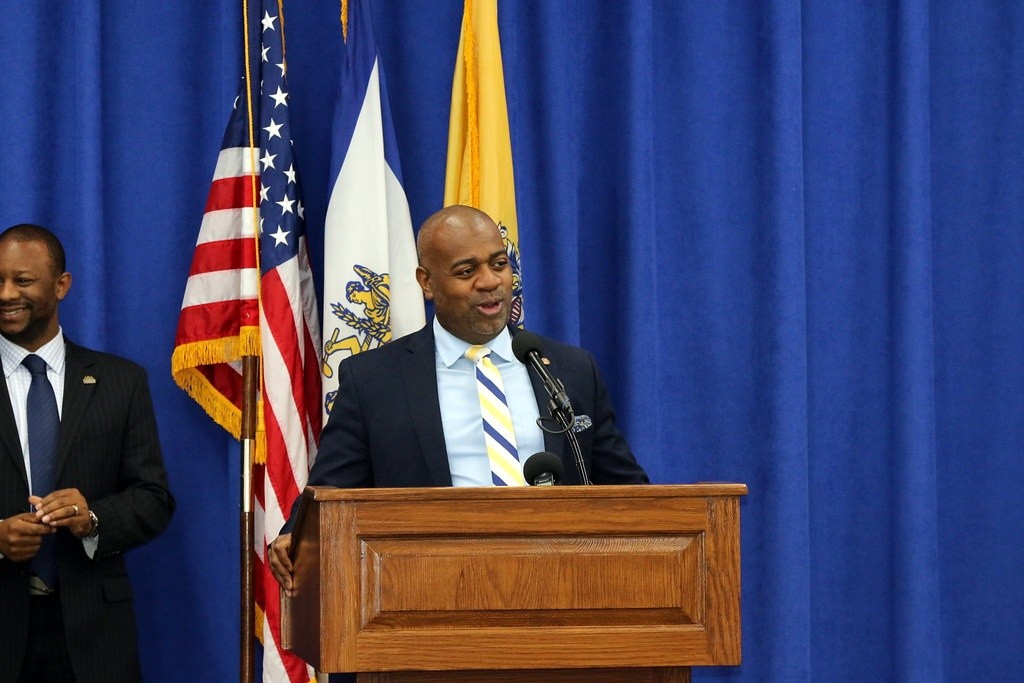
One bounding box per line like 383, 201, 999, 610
72, 505, 78, 516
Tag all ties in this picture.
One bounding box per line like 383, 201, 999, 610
465, 345, 524, 486
20, 354, 62, 591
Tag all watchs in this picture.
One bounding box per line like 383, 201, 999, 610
85, 510, 98, 540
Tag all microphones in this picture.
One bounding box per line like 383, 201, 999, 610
523, 453, 563, 487
511, 331, 576, 416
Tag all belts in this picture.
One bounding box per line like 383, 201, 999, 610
29, 575, 52, 596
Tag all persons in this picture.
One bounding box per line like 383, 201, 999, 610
266, 206, 648, 597
0, 225, 175, 683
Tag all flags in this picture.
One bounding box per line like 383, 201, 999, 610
171, 0, 321, 683
321, 0, 426, 429
443, 0, 525, 332
